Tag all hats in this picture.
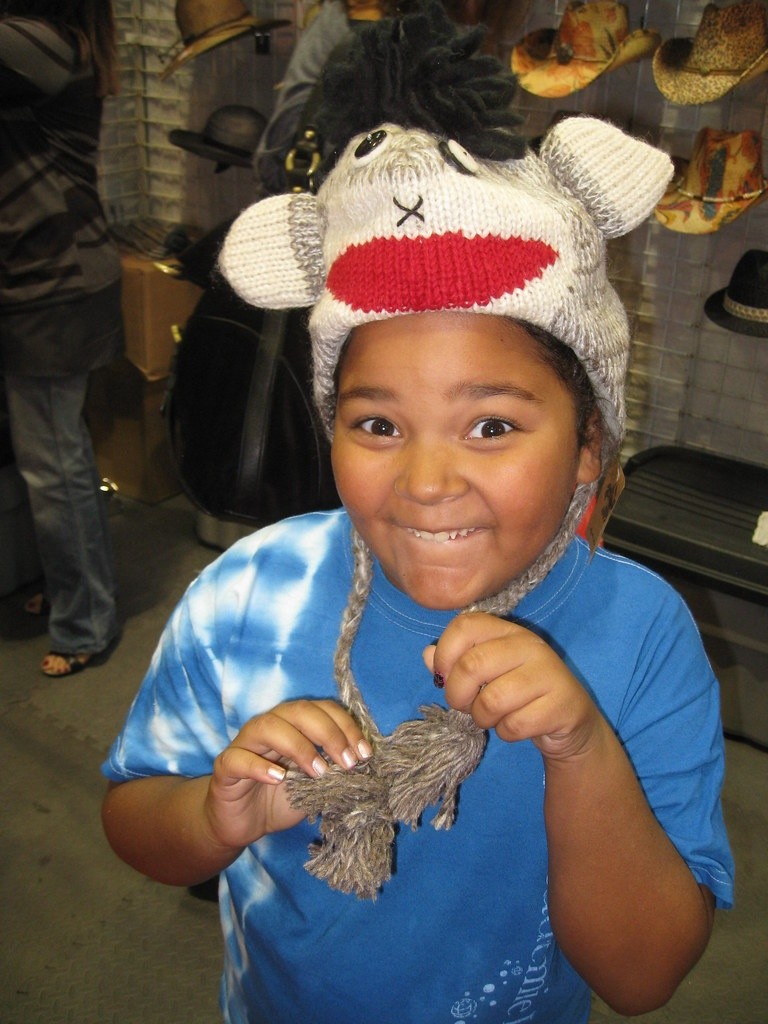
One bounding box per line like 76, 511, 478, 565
705, 249, 768, 338
217, 18, 675, 900
159, 0, 297, 83
510, 0, 662, 98
169, 103, 268, 168
653, 3, 768, 106
653, 127, 768, 235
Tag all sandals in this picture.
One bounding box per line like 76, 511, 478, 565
24, 592, 51, 614
41, 652, 95, 677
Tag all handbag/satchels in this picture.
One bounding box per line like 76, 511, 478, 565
159, 280, 341, 526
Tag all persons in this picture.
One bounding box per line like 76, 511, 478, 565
0, 0, 124, 678
100, 12, 737, 1024
